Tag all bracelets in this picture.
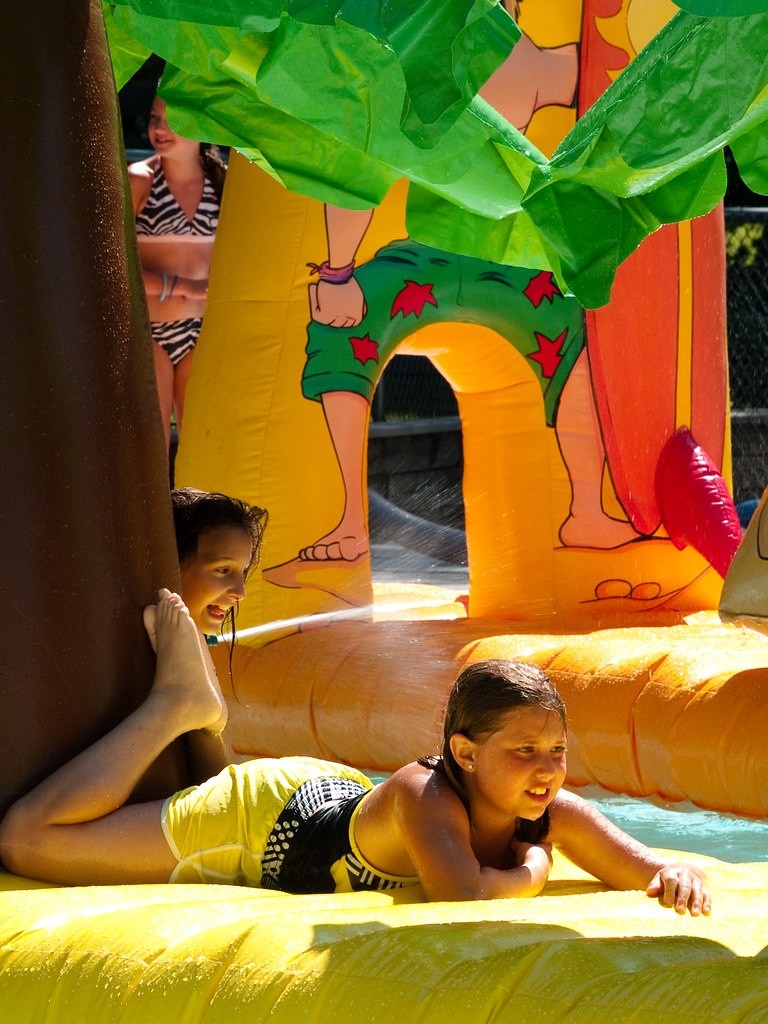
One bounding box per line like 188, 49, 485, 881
156, 273, 178, 302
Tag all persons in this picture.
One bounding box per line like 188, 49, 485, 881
0, 588, 712, 917
171, 486, 270, 704
127, 96, 229, 458
